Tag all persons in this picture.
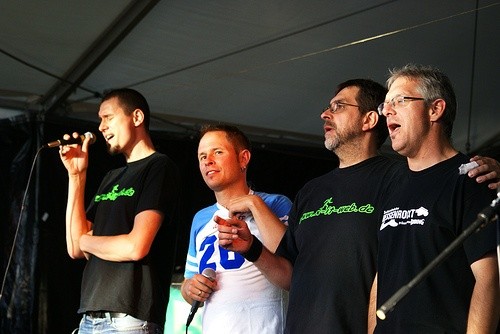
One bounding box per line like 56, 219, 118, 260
214, 79, 500, 334
58, 88, 175, 334
368, 63, 500, 334
180, 122, 293, 334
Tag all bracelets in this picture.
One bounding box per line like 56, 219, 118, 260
239, 235, 262, 261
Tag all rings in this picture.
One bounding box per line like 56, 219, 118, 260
198, 290, 202, 296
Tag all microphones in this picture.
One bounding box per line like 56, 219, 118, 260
47, 132, 96, 147
186, 267, 216, 326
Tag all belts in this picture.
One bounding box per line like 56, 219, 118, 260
86, 312, 127, 318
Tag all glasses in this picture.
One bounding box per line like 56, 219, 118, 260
377, 96, 430, 115
323, 101, 359, 113
